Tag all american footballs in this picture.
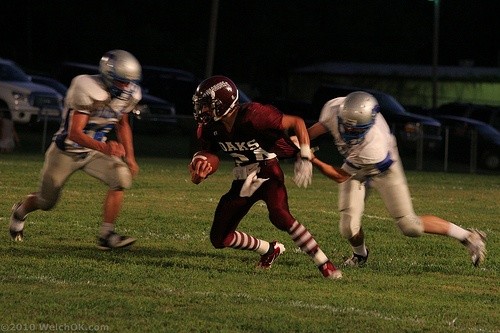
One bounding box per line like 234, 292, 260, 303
191, 151, 220, 175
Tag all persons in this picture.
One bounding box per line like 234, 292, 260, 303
8, 49, 144, 251
297, 92, 488, 269
183, 78, 343, 280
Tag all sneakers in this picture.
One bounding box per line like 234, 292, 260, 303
317, 259, 340, 280
93, 230, 139, 250
10, 200, 23, 241
258, 241, 285, 269
461, 227, 488, 267
345, 250, 369, 266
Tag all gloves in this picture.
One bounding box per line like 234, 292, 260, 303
294, 145, 313, 189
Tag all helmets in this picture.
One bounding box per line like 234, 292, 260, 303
98, 49, 141, 99
336, 90, 379, 144
191, 74, 240, 125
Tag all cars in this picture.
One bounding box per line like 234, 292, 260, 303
434, 114, 500, 169
356, 87, 442, 155
51, 63, 175, 119
0, 61, 65, 124
